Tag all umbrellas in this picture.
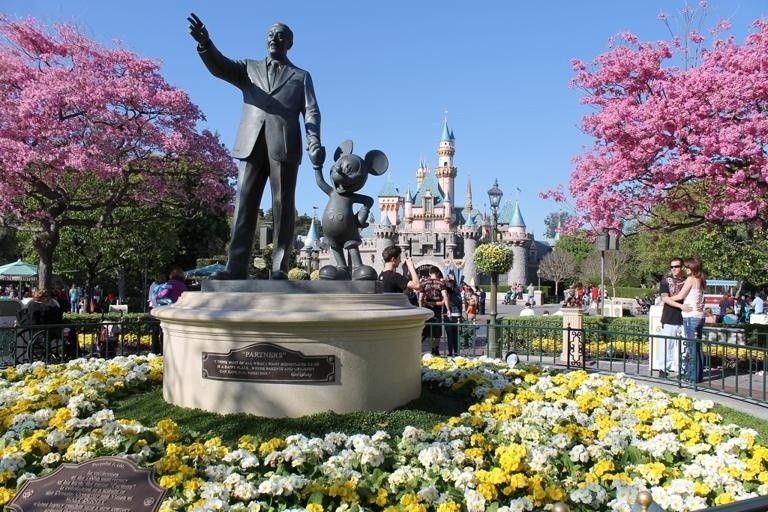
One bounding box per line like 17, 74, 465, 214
0, 258, 38, 299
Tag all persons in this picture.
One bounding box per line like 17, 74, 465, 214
658, 257, 705, 378
560, 283, 608, 308
717, 292, 767, 328
1, 282, 116, 314
518, 303, 534, 316
156, 266, 189, 305
185, 12, 323, 281
704, 307, 716, 324
668, 257, 705, 384
148, 270, 166, 355
378, 245, 421, 294
509, 281, 535, 308
405, 266, 486, 357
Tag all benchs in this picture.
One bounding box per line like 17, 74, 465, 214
607, 296, 643, 317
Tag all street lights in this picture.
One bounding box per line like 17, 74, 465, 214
487, 177, 503, 360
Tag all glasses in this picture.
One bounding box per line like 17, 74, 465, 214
670, 265, 680, 269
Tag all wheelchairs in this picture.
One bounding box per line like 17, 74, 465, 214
16, 303, 69, 364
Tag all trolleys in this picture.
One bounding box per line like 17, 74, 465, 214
86, 308, 125, 359
